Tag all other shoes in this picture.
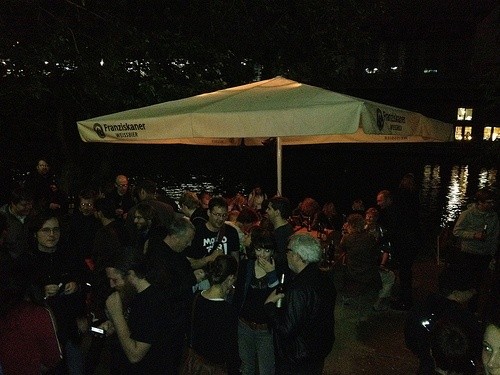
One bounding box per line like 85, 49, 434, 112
373, 303, 390, 311
390, 300, 412, 311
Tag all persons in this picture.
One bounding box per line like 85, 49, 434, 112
1, 155, 394, 375
375, 170, 500, 375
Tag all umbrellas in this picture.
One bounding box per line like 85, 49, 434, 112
76, 75, 455, 195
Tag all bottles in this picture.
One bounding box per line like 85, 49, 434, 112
276, 273, 285, 308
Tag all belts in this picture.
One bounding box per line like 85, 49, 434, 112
239, 317, 268, 330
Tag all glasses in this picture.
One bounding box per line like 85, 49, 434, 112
132, 216, 145, 219
284, 248, 294, 254
210, 210, 228, 217
38, 227, 60, 232
